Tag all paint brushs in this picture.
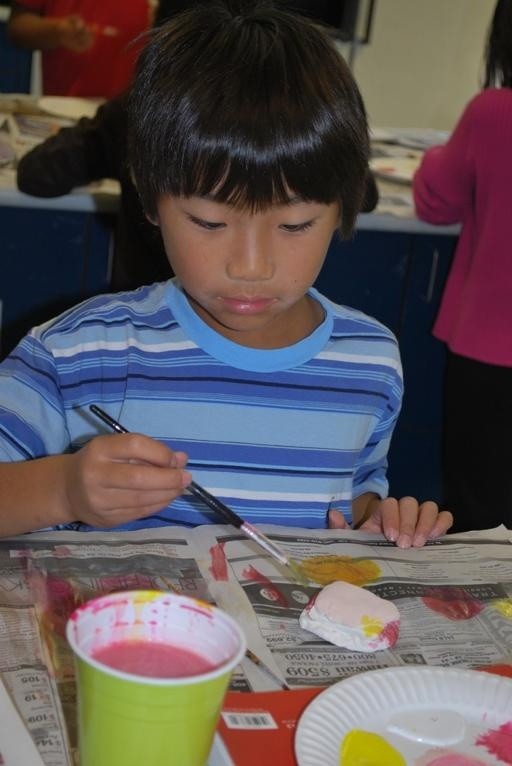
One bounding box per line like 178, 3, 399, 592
89, 405, 309, 589
160, 576, 291, 692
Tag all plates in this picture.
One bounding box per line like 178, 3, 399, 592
293, 665, 512, 765
38, 94, 97, 124
367, 155, 422, 185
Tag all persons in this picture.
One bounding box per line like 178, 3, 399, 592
17, 0, 378, 296
410, 0, 512, 531
0, 1, 457, 549
1, 0, 118, 95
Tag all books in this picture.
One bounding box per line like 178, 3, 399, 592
214, 665, 511, 766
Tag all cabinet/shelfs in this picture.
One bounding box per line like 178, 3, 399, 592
0, 92, 464, 489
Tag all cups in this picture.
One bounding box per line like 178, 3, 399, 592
64, 588, 247, 766
